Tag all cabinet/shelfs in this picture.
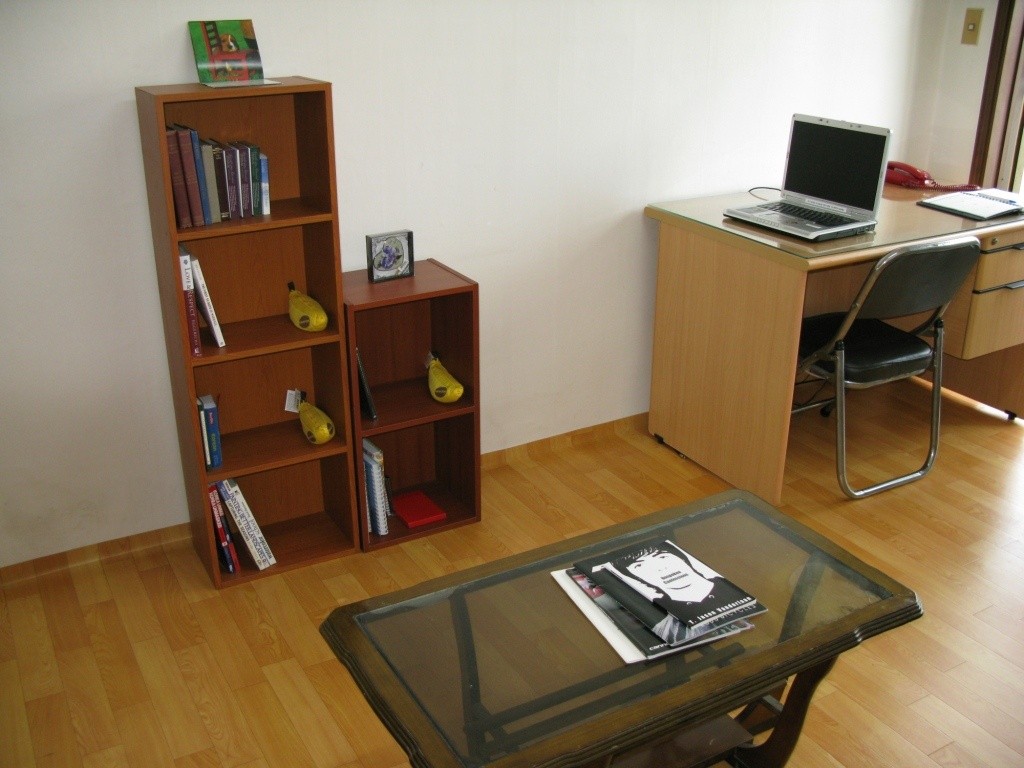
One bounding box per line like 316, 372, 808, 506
340, 257, 501, 555
129, 74, 362, 591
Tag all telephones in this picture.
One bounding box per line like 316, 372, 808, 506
885, 160, 936, 187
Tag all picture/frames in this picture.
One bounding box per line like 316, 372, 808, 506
366, 230, 415, 281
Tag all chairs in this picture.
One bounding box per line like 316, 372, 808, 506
787, 233, 984, 503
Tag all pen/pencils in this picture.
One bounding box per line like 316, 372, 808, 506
978, 192, 1016, 203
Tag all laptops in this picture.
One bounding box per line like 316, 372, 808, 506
723, 113, 893, 243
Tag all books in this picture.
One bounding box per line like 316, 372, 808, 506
362, 437, 392, 536
196, 394, 221, 471
550, 540, 769, 665
179, 246, 226, 357
207, 478, 276, 574
165, 122, 271, 229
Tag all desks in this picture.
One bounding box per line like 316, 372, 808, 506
314, 487, 926, 768
646, 182, 1024, 509
